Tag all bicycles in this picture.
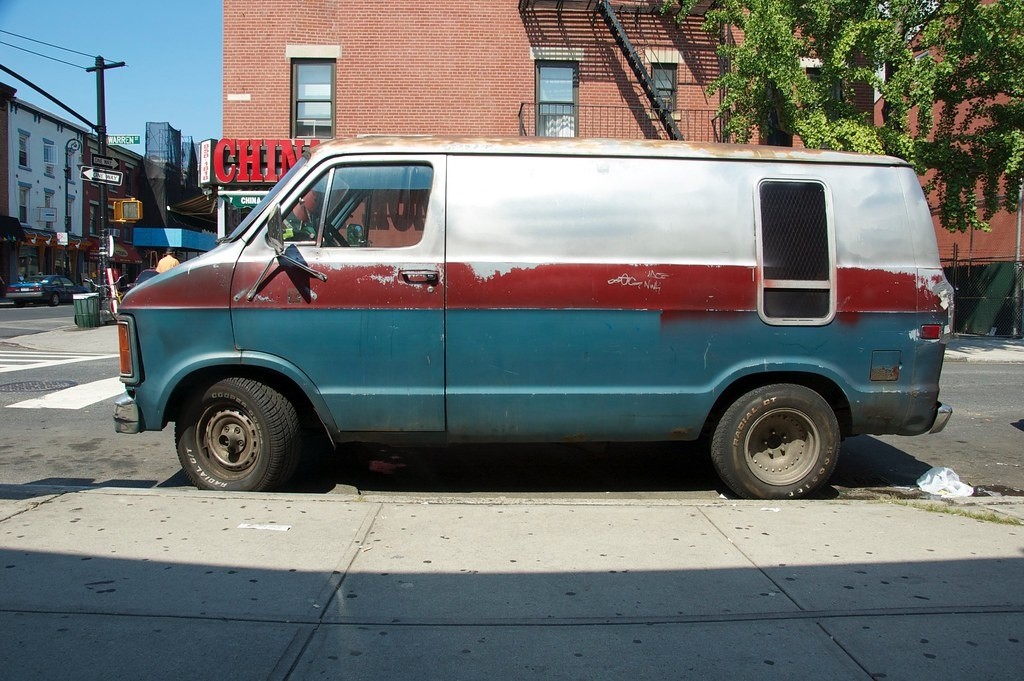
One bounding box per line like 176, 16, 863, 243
83, 273, 130, 322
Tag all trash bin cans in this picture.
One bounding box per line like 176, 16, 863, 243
73, 292, 99, 327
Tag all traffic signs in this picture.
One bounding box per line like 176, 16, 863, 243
90, 153, 120, 171
78, 166, 124, 189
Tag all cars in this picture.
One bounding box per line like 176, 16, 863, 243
6, 274, 92, 307
126, 269, 160, 292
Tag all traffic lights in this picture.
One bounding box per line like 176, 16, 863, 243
114, 202, 123, 222
123, 202, 142, 220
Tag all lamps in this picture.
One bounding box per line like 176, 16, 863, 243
69, 237, 82, 248
23, 229, 52, 245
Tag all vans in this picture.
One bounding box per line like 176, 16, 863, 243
110, 133, 956, 501
